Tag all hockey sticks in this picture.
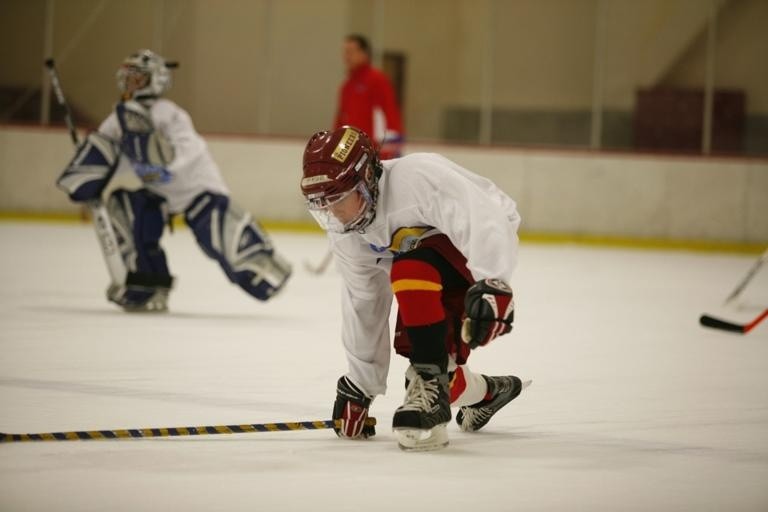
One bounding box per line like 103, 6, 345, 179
699, 308, 768, 332
42, 59, 153, 302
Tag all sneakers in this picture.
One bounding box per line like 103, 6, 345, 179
457, 373, 521, 431
393, 361, 452, 429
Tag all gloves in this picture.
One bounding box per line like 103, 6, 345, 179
332, 375, 371, 438
464, 276, 513, 346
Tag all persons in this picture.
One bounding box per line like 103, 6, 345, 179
328, 32, 405, 161
56, 47, 292, 308
300, 124, 521, 449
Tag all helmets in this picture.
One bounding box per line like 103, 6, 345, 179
301, 125, 382, 234
117, 49, 174, 101
